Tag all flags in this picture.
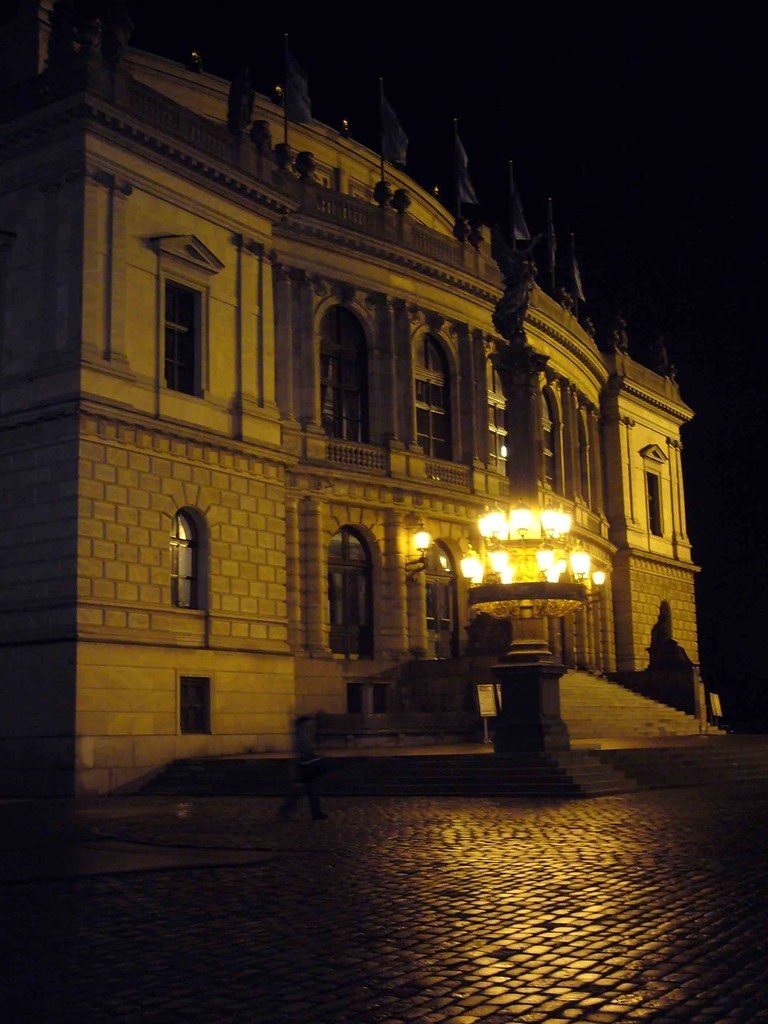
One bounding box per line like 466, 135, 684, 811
225, 21, 597, 324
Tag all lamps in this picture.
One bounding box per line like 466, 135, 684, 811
405, 519, 431, 583
587, 567, 607, 604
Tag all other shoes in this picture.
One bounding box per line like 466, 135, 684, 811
312, 813, 329, 822
276, 816, 293, 824
